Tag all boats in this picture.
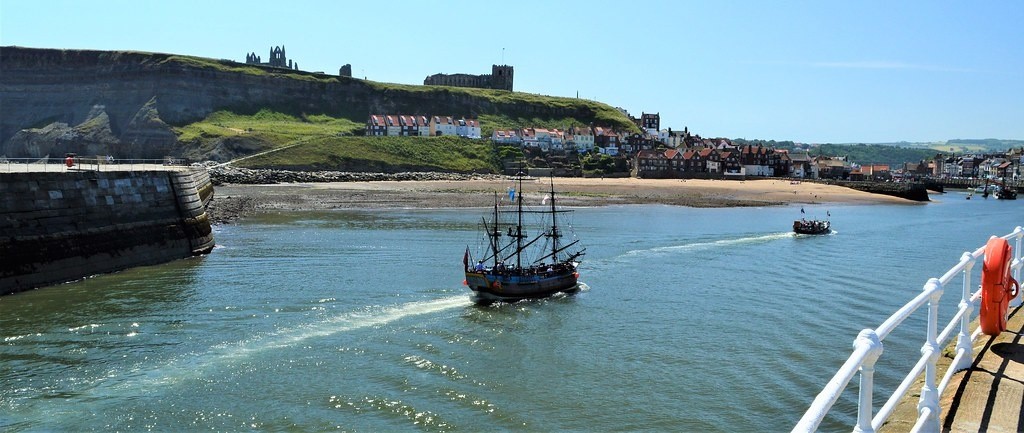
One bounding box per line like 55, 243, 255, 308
993, 175, 1020, 202
463, 159, 588, 304
975, 185, 998, 194
792, 208, 832, 235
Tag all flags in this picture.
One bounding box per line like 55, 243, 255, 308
801, 208, 805, 213
508, 189, 519, 202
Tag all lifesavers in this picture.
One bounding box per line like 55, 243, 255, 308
979, 237, 1012, 337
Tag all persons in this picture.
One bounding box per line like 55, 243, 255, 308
801, 220, 829, 229
472, 261, 571, 275
